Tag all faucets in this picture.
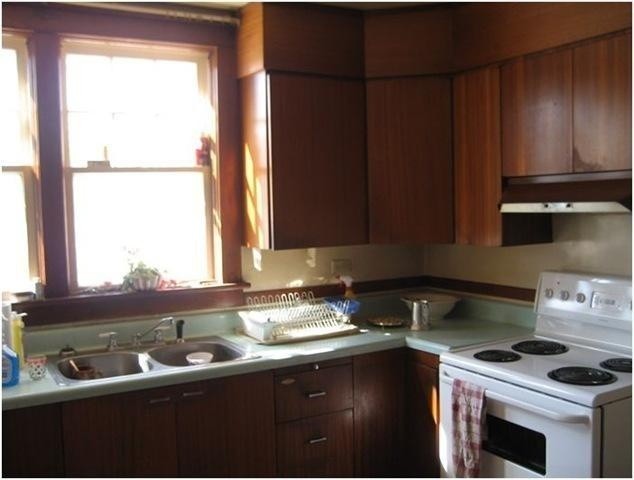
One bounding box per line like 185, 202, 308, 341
136, 316, 174, 344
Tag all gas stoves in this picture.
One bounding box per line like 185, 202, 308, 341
440, 330, 633, 406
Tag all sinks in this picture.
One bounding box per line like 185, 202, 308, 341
148, 336, 262, 370
45, 350, 154, 385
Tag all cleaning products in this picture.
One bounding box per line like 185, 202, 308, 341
336, 275, 355, 299
2, 312, 20, 387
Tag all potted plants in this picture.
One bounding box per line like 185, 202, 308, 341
123, 262, 162, 292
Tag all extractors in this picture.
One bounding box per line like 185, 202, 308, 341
498, 181, 631, 214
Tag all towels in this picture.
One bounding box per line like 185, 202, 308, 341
452, 377, 489, 478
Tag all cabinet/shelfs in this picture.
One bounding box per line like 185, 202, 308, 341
454, 63, 555, 248
266, 72, 368, 251
2, 347, 440, 478
499, 31, 631, 178
368, 74, 456, 245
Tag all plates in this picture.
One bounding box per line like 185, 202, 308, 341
367, 312, 409, 327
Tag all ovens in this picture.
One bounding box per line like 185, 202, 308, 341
437, 363, 634, 477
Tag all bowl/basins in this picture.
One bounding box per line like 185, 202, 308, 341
185, 352, 213, 364
123, 276, 161, 292
400, 293, 462, 321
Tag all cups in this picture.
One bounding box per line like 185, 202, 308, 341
410, 300, 433, 332
72, 366, 103, 380
27, 354, 47, 380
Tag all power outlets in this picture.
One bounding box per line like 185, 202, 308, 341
331, 259, 352, 272
